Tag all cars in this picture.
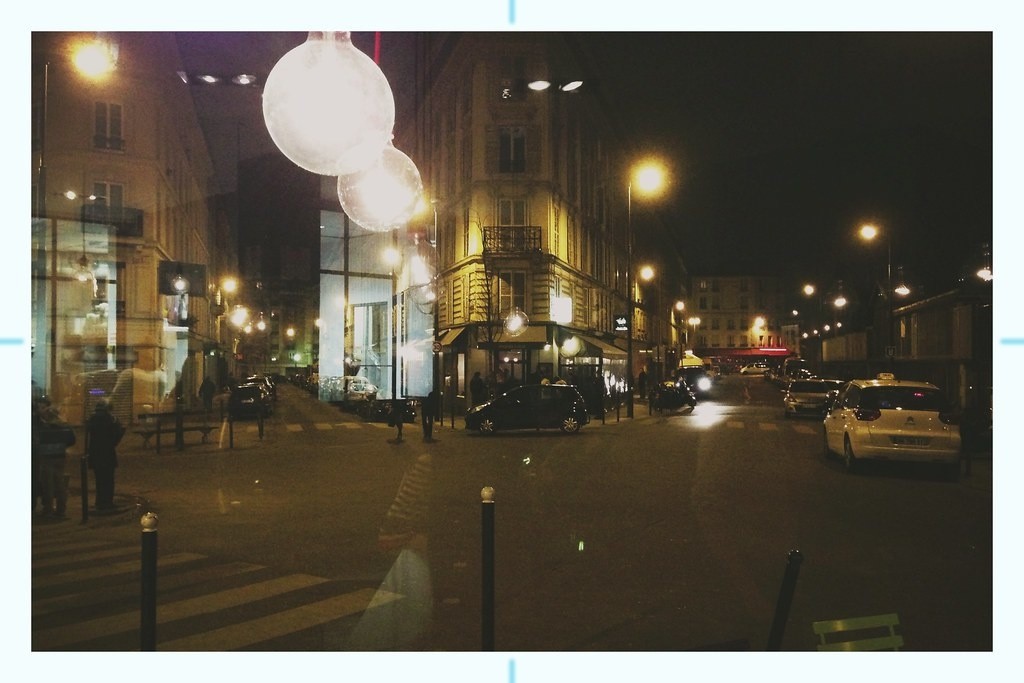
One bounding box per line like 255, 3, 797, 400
229, 374, 276, 419
463, 379, 591, 437
289, 373, 418, 424
778, 357, 842, 416
741, 364, 772, 375
683, 369, 714, 398
826, 373, 962, 472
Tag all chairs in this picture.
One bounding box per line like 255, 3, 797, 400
50, 370, 165, 423
812, 614, 904, 651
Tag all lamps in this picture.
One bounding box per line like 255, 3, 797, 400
74, 198, 91, 280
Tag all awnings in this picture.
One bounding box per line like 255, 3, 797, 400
559, 329, 628, 359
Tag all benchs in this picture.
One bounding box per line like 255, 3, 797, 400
132, 409, 220, 449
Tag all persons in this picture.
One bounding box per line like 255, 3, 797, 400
199, 377, 216, 413
85, 397, 127, 511
638, 369, 647, 398
32, 395, 75, 516
422, 400, 433, 439
470, 368, 566, 406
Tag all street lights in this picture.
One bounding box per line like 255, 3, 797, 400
625, 172, 659, 419
674, 299, 686, 361
803, 283, 824, 360
858, 223, 893, 369
